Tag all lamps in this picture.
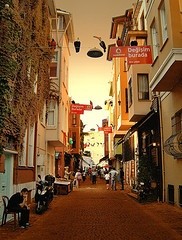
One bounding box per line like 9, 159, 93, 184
68, 138, 73, 145
87, 47, 103, 58
95, 105, 102, 109
31, 39, 56, 50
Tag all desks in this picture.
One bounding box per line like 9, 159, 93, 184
54, 180, 72, 196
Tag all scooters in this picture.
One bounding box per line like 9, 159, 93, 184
93, 175, 97, 184
35, 174, 55, 214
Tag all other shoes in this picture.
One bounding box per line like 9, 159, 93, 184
26, 223, 32, 227
18, 226, 24, 228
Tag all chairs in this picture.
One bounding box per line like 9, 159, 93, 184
1, 196, 19, 227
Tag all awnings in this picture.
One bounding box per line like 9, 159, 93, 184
100, 155, 108, 162
116, 109, 156, 145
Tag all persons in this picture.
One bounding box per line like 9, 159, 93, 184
119, 168, 124, 190
96, 167, 108, 179
105, 171, 110, 189
109, 168, 118, 191
75, 170, 81, 186
64, 167, 71, 180
88, 168, 96, 184
8, 188, 32, 228
81, 170, 86, 181
